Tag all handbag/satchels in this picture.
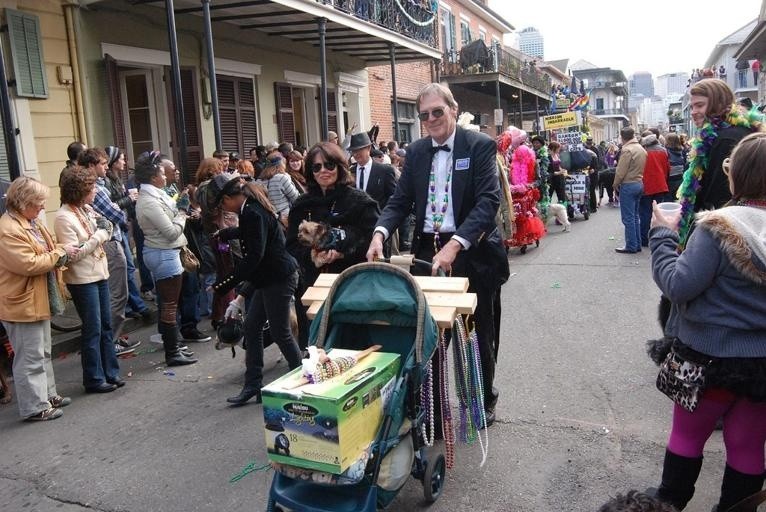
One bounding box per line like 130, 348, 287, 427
656, 351, 708, 413
180, 249, 200, 273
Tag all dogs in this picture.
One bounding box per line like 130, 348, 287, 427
297, 219, 347, 268
0, 341, 12, 404
214, 300, 299, 364
537, 200, 571, 233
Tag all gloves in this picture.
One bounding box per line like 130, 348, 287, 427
223, 300, 239, 324
178, 193, 191, 213
96, 218, 111, 231
92, 227, 109, 247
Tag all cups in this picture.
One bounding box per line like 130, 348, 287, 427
127, 186, 139, 203
656, 201, 685, 222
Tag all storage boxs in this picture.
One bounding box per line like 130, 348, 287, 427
259, 347, 403, 475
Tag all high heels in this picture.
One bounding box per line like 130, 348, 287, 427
227, 383, 261, 402
211, 320, 225, 331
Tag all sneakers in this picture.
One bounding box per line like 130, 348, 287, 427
114, 343, 135, 356
117, 336, 141, 348
26, 407, 63, 421
50, 395, 71, 407
142, 290, 155, 301
399, 241, 412, 251
181, 323, 211, 342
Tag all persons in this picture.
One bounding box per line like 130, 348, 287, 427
366, 83, 510, 430
492, 127, 622, 242
51, 129, 407, 407
0, 177, 81, 418
611, 64, 766, 510
528, 59, 569, 98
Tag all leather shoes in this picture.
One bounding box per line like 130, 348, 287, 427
615, 247, 637, 253
127, 310, 145, 321
105, 375, 124, 387
85, 382, 118, 392
141, 307, 157, 323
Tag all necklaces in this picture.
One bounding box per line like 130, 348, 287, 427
418, 314, 488, 469
428, 161, 452, 254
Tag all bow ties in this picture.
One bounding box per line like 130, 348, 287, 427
432, 144, 450, 154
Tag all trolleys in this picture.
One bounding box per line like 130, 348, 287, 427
503, 187, 543, 255
565, 165, 593, 220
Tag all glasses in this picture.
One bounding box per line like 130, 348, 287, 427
417, 110, 443, 121
311, 162, 336, 172
28, 199, 46, 206
722, 158, 731, 176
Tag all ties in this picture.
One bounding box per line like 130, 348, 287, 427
360, 167, 364, 189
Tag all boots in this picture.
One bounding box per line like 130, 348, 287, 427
180, 349, 194, 357
161, 325, 198, 366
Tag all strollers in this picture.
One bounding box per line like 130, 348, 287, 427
261, 257, 446, 511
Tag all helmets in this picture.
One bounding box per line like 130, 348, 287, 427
206, 173, 240, 208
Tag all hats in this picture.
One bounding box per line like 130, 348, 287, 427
371, 150, 383, 156
586, 138, 594, 144
346, 132, 374, 150
103, 145, 124, 168
229, 151, 240, 161
